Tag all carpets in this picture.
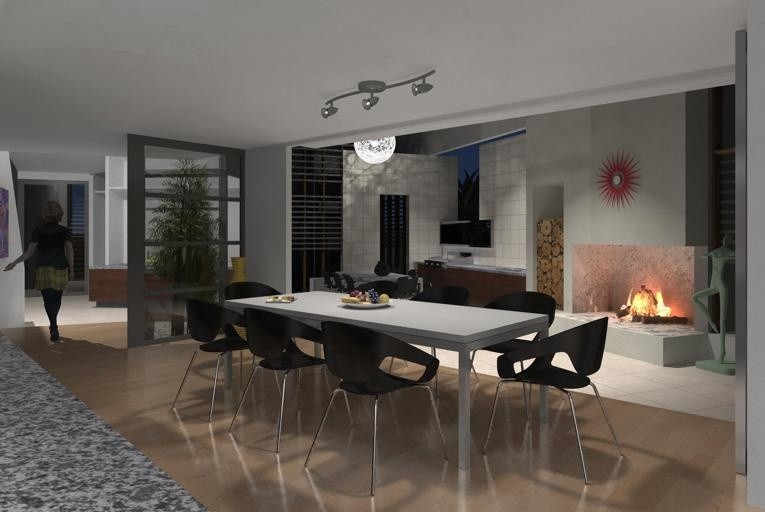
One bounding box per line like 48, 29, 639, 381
0, 328, 206, 512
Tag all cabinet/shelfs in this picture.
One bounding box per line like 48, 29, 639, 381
416, 264, 526, 306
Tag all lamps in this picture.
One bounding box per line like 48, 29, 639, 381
362, 92, 379, 111
353, 136, 397, 165
411, 77, 434, 96
320, 101, 338, 119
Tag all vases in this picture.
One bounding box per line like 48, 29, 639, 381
230, 255, 248, 285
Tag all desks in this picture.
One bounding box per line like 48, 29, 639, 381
335, 271, 410, 288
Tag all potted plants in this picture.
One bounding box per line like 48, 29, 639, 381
142, 158, 225, 336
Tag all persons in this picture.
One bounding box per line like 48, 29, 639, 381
3, 201, 74, 344
690, 233, 736, 364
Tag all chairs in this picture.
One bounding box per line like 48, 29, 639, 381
331, 272, 347, 293
342, 274, 355, 294
322, 270, 336, 288
409, 285, 476, 396
482, 315, 624, 484
227, 306, 337, 453
304, 319, 449, 496
170, 298, 282, 422
224, 281, 283, 384
470, 291, 557, 391
358, 280, 401, 371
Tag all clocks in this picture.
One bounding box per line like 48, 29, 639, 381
593, 148, 643, 210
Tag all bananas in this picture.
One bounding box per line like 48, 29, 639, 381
341, 296, 362, 303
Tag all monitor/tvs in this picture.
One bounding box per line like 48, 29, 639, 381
439, 219, 496, 268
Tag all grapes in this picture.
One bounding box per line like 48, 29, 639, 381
368, 289, 378, 304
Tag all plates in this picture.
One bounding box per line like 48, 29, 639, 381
337, 301, 388, 309
